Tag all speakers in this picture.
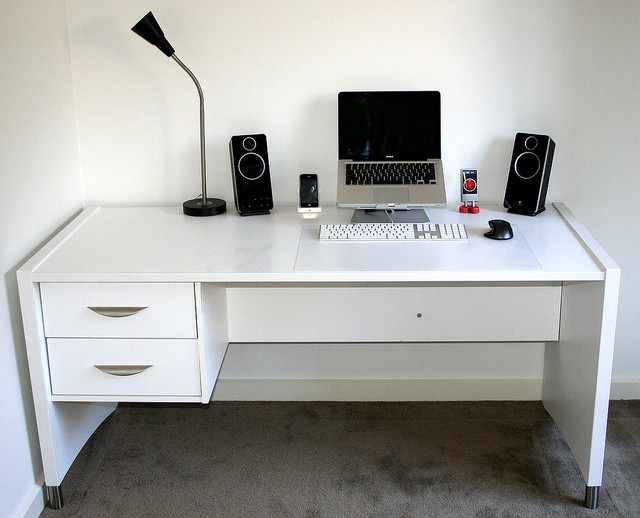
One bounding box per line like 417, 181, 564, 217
228, 133, 273, 216
503, 132, 555, 218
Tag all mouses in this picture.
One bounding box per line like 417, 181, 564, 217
484, 220, 513, 239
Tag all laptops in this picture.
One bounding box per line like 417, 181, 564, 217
335, 91, 447, 208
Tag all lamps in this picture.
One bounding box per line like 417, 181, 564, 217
128, 9, 227, 217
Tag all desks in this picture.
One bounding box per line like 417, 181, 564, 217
14, 202, 623, 511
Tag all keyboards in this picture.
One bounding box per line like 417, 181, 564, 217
318, 223, 468, 241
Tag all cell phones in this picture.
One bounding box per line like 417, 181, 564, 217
300, 173, 318, 208
